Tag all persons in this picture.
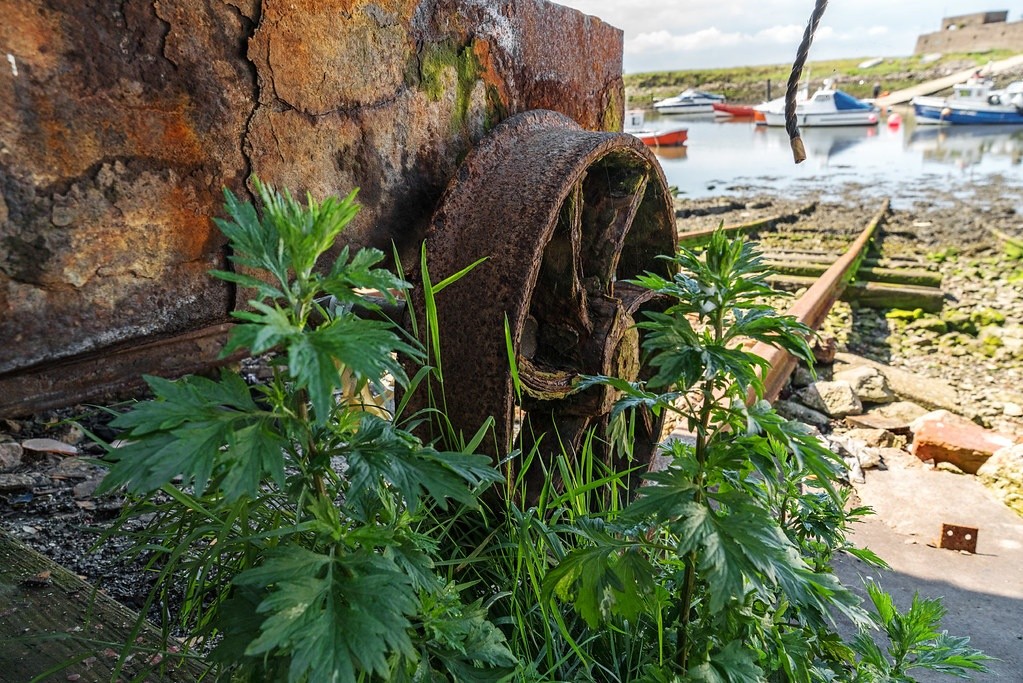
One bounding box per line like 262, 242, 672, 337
872, 81, 882, 98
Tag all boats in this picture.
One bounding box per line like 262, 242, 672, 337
910, 68, 1023, 126
752, 88, 809, 125
712, 102, 754, 118
654, 88, 726, 114
764, 88, 879, 128
623, 108, 689, 147
